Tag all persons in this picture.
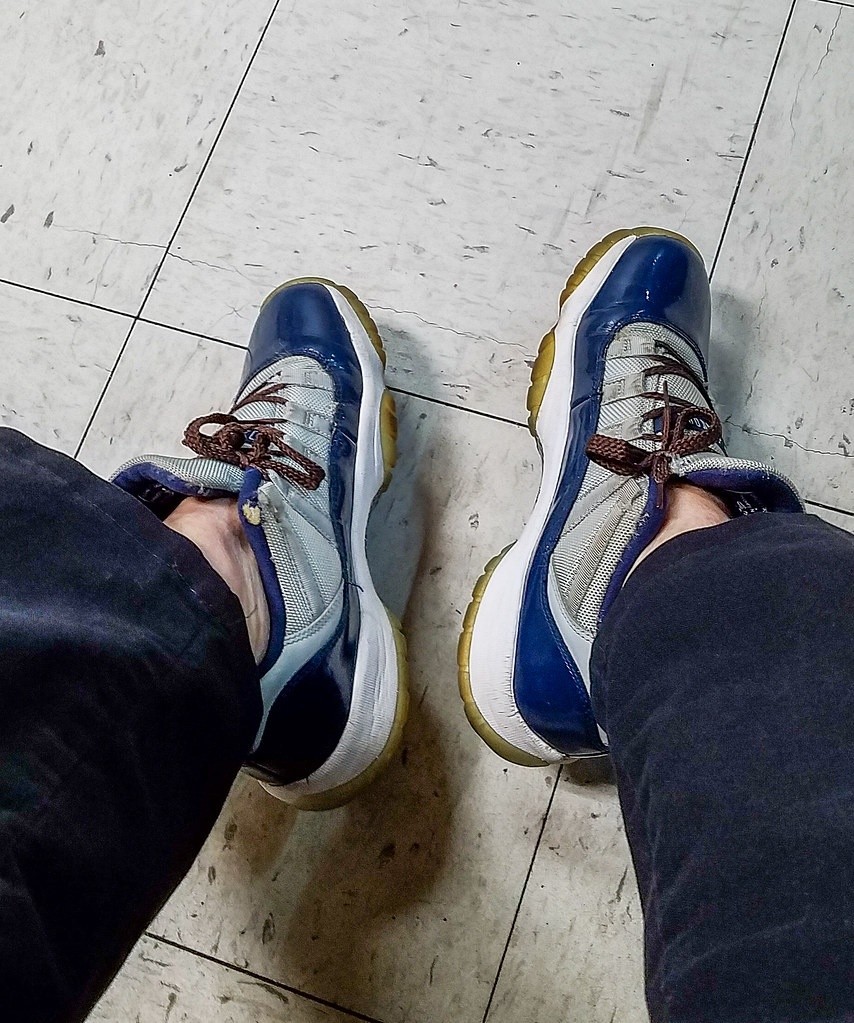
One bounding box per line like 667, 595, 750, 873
0, 224, 854, 1023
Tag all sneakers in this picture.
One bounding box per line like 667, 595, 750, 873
455, 229, 805, 771
104, 280, 415, 811
